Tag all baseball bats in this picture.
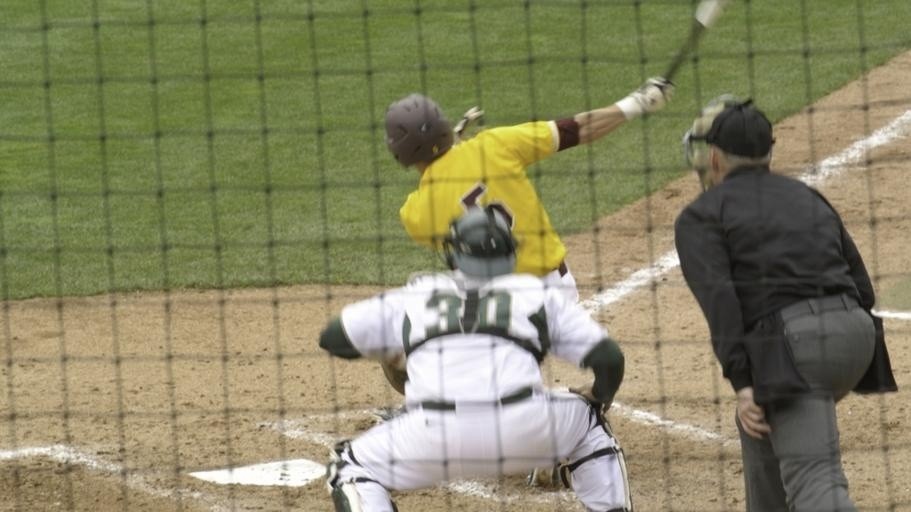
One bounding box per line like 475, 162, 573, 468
663, 1, 724, 82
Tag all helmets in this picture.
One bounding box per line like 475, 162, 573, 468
385, 93, 454, 167
445, 209, 518, 275
682, 99, 775, 192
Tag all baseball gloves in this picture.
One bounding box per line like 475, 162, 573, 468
381, 350, 407, 397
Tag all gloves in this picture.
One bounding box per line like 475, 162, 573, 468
454, 105, 484, 137
631, 75, 677, 112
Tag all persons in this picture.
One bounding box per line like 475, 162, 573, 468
672, 94, 901, 512
316, 209, 637, 511
380, 73, 679, 304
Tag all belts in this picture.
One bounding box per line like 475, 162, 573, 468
558, 260, 567, 277
781, 293, 859, 323
422, 388, 532, 410
